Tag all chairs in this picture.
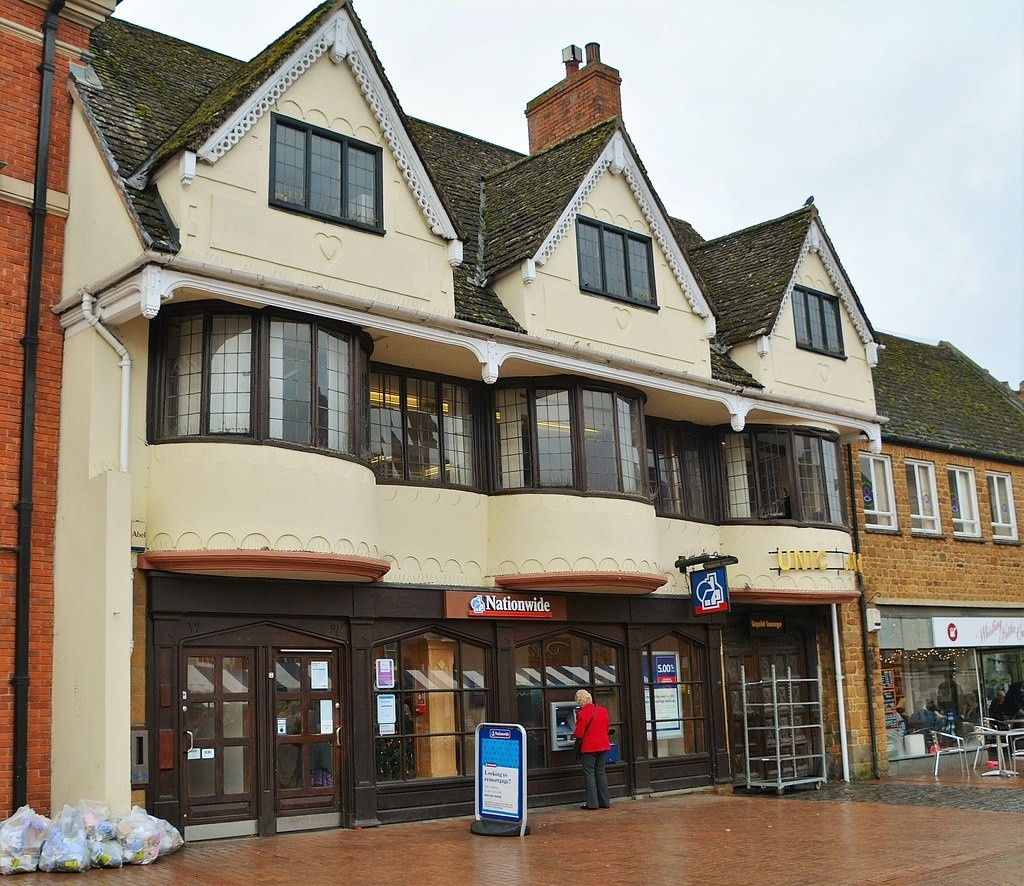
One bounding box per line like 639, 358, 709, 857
909, 716, 1024, 778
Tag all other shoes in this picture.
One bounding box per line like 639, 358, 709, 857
581, 806, 599, 810
600, 807, 611, 809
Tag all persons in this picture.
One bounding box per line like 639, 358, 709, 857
574, 690, 610, 810
897, 702, 943, 753
989, 692, 1006, 730
961, 695, 978, 724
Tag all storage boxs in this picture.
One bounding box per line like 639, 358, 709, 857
603, 744, 618, 764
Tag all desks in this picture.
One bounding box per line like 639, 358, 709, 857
969, 731, 1021, 777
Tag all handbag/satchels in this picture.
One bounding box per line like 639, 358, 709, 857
567, 738, 582, 761
